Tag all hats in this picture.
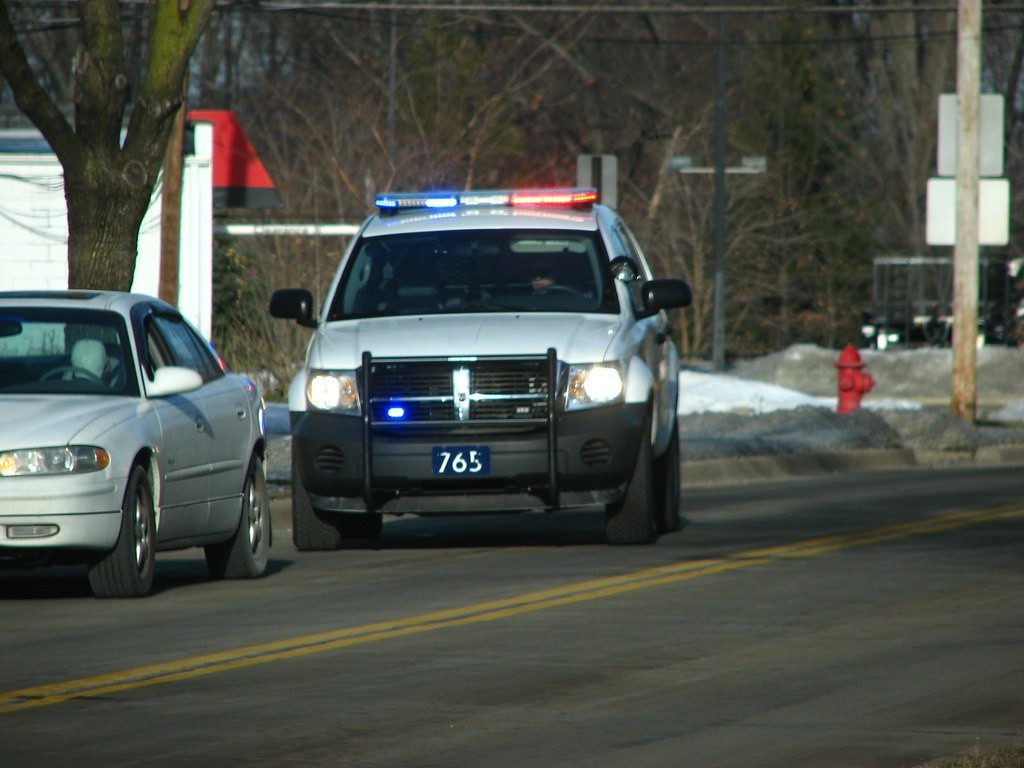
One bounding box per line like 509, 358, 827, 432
72, 340, 106, 381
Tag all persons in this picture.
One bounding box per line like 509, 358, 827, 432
530, 257, 560, 290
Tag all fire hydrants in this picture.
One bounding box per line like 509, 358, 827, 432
833, 343, 877, 417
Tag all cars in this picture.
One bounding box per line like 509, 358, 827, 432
0, 290, 273, 596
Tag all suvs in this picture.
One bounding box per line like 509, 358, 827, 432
269, 192, 693, 545
